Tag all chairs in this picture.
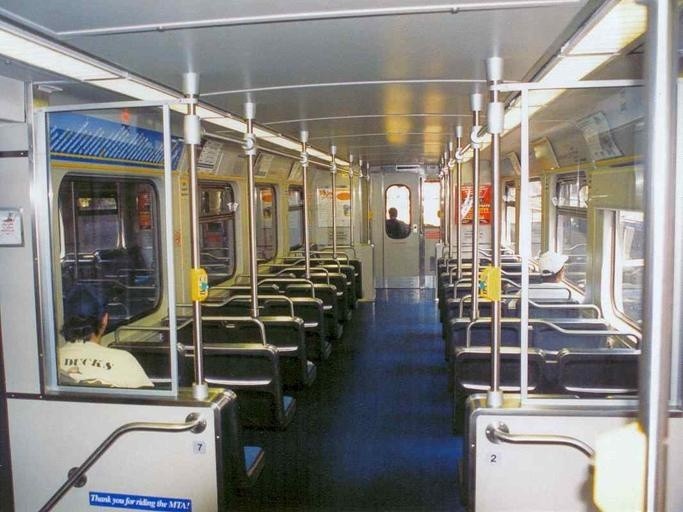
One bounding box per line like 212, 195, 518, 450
228, 244, 362, 345
158, 294, 318, 400
199, 278, 335, 364
443, 292, 611, 367
105, 315, 298, 434
435, 239, 581, 324
428, 237, 642, 512
451, 316, 642, 434
62, 382, 270, 496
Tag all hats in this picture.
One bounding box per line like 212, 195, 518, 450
62, 284, 109, 331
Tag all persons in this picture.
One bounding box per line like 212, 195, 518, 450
54, 281, 155, 388
538, 249, 569, 283
384, 207, 410, 240
